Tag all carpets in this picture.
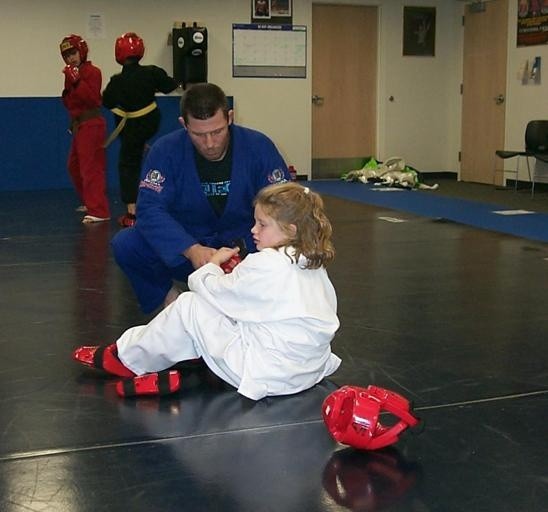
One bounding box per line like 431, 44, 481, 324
303, 179, 548, 243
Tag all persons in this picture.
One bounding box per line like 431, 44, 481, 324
72, 181, 342, 402
107, 81, 294, 315
99, 32, 180, 228
57, 32, 114, 226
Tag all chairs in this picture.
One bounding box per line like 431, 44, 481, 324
494, 119, 548, 198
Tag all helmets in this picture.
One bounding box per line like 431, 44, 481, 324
115, 31, 145, 65
322, 384, 419, 451
59, 34, 88, 67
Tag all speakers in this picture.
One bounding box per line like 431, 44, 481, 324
172, 27, 208, 85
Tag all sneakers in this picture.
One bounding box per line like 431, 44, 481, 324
72, 344, 135, 380
118, 216, 135, 226
116, 368, 181, 397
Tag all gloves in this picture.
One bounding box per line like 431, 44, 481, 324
62, 64, 80, 85
